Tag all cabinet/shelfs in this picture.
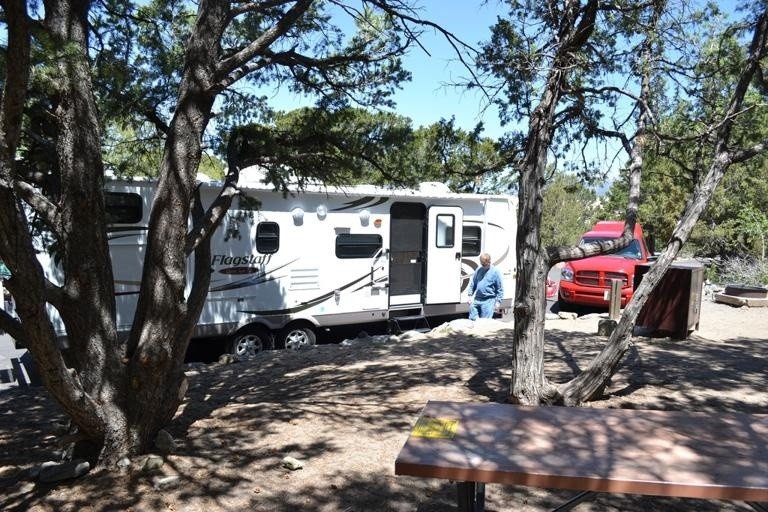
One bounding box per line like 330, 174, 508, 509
633, 265, 704, 340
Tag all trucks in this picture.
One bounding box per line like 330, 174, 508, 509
555, 223, 646, 318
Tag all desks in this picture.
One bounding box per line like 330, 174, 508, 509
395, 401, 768, 512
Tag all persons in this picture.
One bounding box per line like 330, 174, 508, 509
467, 252, 503, 321
